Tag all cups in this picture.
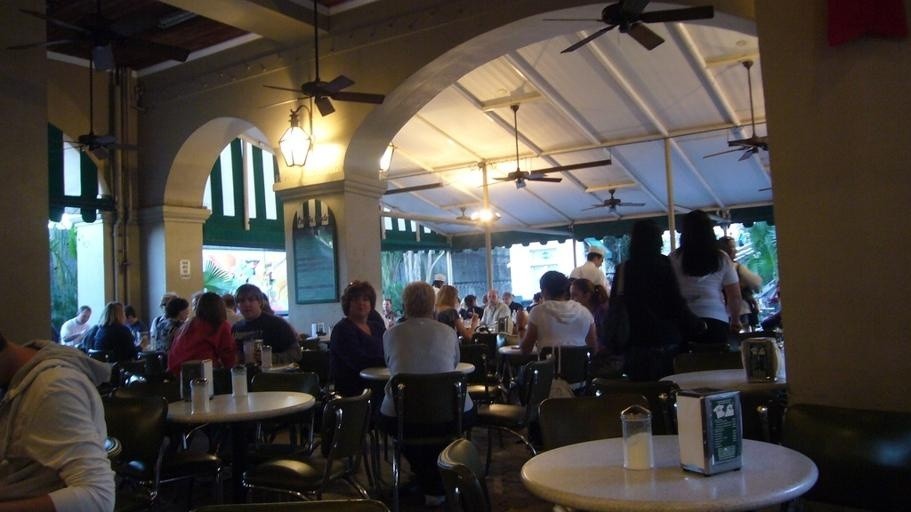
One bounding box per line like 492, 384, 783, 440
137, 329, 149, 342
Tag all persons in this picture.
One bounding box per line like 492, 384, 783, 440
58, 285, 305, 507
326, 280, 524, 507
0, 336, 119, 512
519, 247, 612, 454
612, 208, 761, 436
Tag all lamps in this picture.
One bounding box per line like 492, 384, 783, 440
379, 142, 396, 173
279, 106, 313, 168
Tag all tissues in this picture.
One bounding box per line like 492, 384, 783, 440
176, 358, 215, 405
675, 386, 745, 477
740, 337, 781, 380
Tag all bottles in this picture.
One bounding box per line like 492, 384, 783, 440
231, 365, 249, 398
621, 404, 653, 473
189, 378, 210, 414
516, 305, 530, 342
261, 346, 274, 367
252, 339, 264, 361
150, 334, 157, 350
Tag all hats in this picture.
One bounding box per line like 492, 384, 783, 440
435, 274, 446, 281
589, 247, 604, 257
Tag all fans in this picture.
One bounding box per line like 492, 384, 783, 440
265, 0, 386, 117
6, 0, 191, 71
494, 105, 564, 190
64, 51, 138, 160
541, 0, 715, 54
580, 187, 646, 218
703, 61, 768, 164
